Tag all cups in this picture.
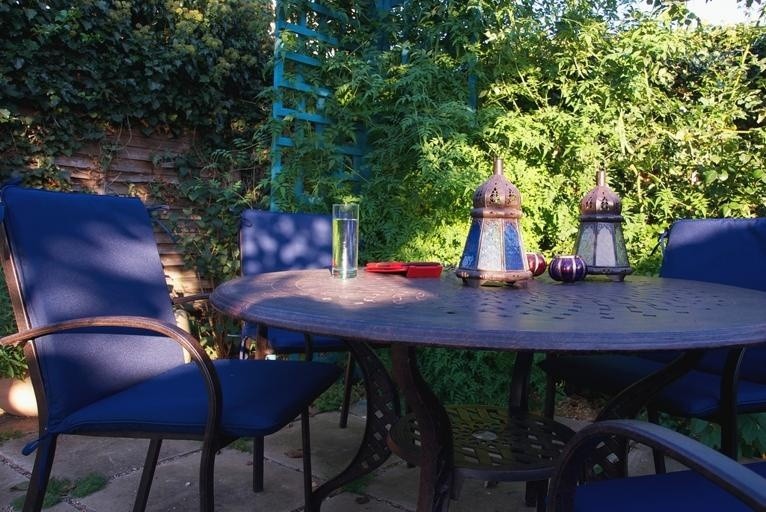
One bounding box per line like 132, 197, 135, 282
331, 203, 360, 279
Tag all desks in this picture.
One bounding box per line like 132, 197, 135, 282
203, 263, 764, 510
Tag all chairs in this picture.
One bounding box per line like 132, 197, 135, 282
5, 190, 347, 512
540, 217, 761, 464
546, 415, 763, 507
230, 208, 423, 494
